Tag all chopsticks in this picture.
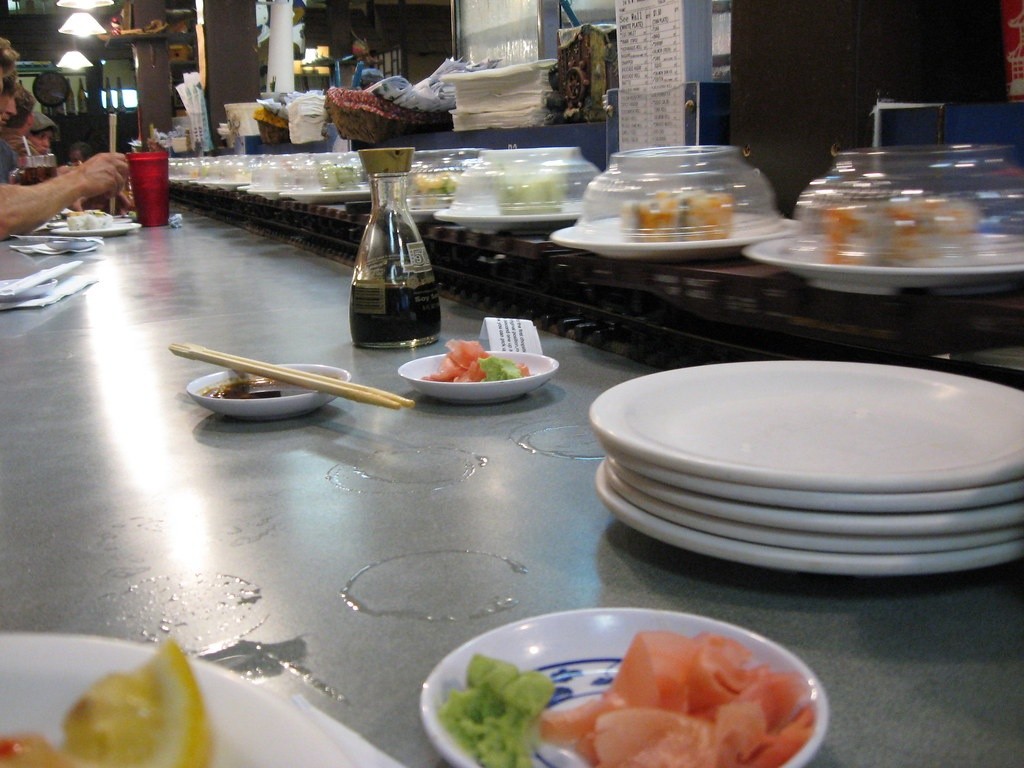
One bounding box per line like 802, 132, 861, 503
168, 341, 416, 409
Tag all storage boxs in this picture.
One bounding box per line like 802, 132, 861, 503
224, 102, 261, 136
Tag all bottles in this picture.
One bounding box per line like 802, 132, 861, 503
111, 18, 121, 36
350, 146, 441, 350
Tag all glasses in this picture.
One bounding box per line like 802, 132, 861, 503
1, 76, 17, 97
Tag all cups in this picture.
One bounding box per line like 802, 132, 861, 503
128, 152, 169, 227
10, 154, 57, 188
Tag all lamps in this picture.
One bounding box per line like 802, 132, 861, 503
56, 36, 94, 70
58, 8, 107, 37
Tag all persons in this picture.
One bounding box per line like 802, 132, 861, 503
0, 37, 131, 240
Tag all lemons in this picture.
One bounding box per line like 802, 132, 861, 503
0, 639, 214, 768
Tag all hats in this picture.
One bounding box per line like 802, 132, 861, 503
29, 111, 59, 132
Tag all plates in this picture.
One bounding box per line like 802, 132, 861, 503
432, 204, 595, 234
585, 360, 1024, 577
0, 623, 408, 768
742, 224, 1023, 297
185, 364, 351, 419
549, 213, 804, 263
166, 174, 375, 206
46, 218, 142, 236
398, 351, 560, 403
402, 198, 445, 225
419, 607, 830, 768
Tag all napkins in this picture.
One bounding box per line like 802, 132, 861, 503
287, 93, 331, 144
9, 244, 98, 255
33, 223, 50, 232
0, 274, 95, 310
439, 60, 558, 132
